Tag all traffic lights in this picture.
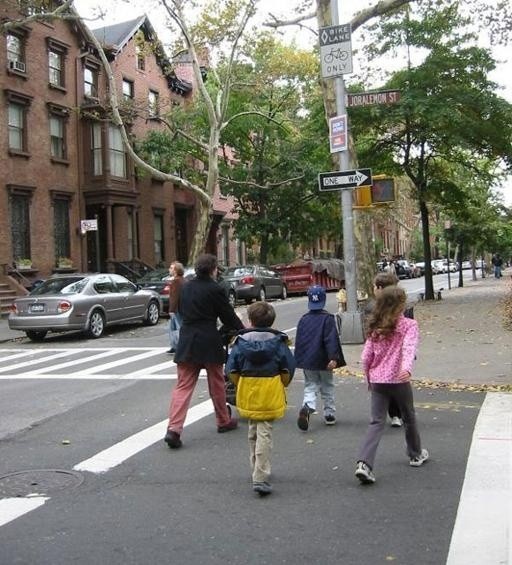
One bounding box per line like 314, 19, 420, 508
367, 175, 399, 205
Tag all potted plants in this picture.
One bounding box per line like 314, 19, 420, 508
16, 257, 32, 270
58, 257, 73, 268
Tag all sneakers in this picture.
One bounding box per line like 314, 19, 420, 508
166, 348, 176, 355
407, 448, 429, 467
354, 461, 376, 483
297, 407, 309, 431
253, 482, 273, 495
217, 418, 237, 433
164, 431, 182, 449
391, 416, 404, 426
325, 416, 336, 425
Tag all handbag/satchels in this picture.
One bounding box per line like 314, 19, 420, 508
167, 313, 182, 345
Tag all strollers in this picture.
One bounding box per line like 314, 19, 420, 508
218, 329, 238, 422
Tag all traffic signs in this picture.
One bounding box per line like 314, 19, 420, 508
346, 90, 401, 108
319, 169, 371, 191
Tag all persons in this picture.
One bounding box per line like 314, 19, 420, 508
491, 253, 503, 279
224, 301, 297, 494
293, 285, 347, 431
355, 286, 429, 483
384, 260, 397, 277
166, 261, 185, 353
364, 272, 413, 427
165, 253, 247, 449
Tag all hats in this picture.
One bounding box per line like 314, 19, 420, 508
307, 285, 326, 310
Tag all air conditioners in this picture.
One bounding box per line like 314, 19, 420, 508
9, 61, 26, 74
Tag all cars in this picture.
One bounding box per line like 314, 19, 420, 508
7, 273, 163, 339
221, 265, 289, 301
139, 271, 238, 312
378, 256, 486, 280
